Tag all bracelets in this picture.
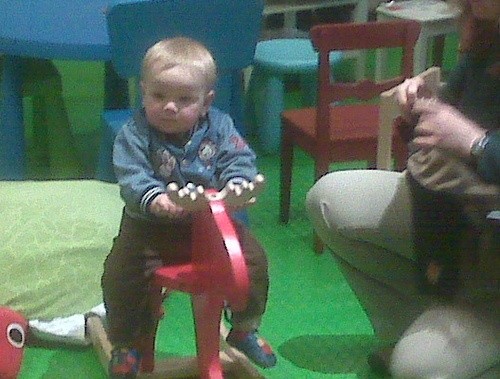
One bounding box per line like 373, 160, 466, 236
470, 129, 497, 168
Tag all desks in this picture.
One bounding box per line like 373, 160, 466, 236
0, 0, 130, 182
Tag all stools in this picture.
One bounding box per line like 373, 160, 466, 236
376, 0, 464, 81
246, 39, 341, 155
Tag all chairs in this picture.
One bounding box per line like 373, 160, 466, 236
377, 66, 440, 169
279, 21, 422, 255
23, 61, 84, 179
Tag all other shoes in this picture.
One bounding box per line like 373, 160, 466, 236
107, 343, 142, 379
225, 329, 277, 369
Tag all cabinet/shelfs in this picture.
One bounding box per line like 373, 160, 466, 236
263, 0, 370, 82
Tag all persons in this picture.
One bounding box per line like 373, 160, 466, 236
304, 0, 500, 379
101, 36, 278, 378
